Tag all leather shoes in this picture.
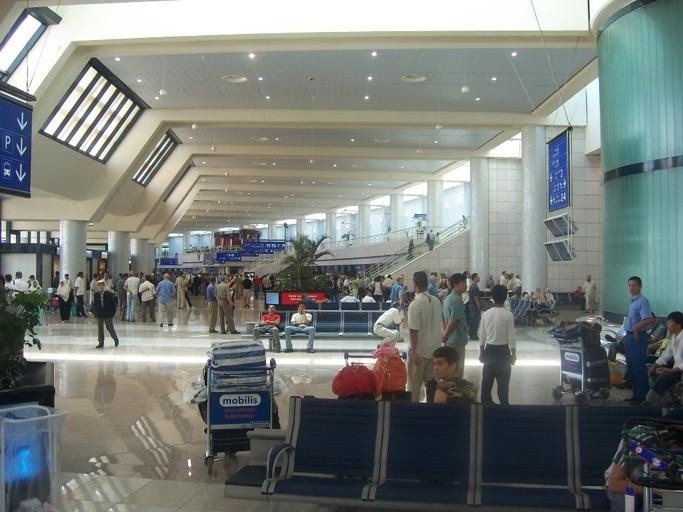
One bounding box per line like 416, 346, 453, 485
605, 335, 616, 342
613, 381, 649, 406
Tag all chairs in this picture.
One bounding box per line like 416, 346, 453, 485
342, 302, 359, 311
508, 299, 530, 318
552, 291, 585, 305
323, 301, 339, 310
363, 303, 378, 311
256, 310, 399, 353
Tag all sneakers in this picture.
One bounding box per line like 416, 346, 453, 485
96, 345, 104, 348
210, 330, 240, 334
306, 349, 314, 352
115, 340, 118, 346
284, 349, 293, 351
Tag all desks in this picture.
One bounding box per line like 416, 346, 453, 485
1, 361, 55, 409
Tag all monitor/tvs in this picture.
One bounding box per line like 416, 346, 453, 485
553, 213, 578, 236
553, 239, 576, 261
544, 241, 562, 261
542, 216, 564, 237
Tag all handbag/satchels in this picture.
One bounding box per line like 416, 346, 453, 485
550, 321, 601, 344
195, 340, 281, 442
332, 365, 377, 398
627, 440, 683, 490
374, 354, 407, 392
649, 365, 681, 396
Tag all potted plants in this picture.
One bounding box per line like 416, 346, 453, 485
0, 287, 48, 390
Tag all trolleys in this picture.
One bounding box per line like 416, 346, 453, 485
204, 341, 281, 475
550, 321, 610, 403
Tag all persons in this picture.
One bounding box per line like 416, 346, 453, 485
424, 346, 477, 402
461, 213, 469, 230
0, 268, 277, 350
602, 380, 682, 512
283, 302, 316, 353
642, 311, 683, 404
424, 227, 441, 251
573, 286, 585, 310
327, 267, 556, 347
584, 274, 597, 314
608, 311, 666, 362
407, 237, 415, 259
405, 271, 443, 403
623, 276, 654, 405
252, 303, 282, 353
478, 285, 517, 407
